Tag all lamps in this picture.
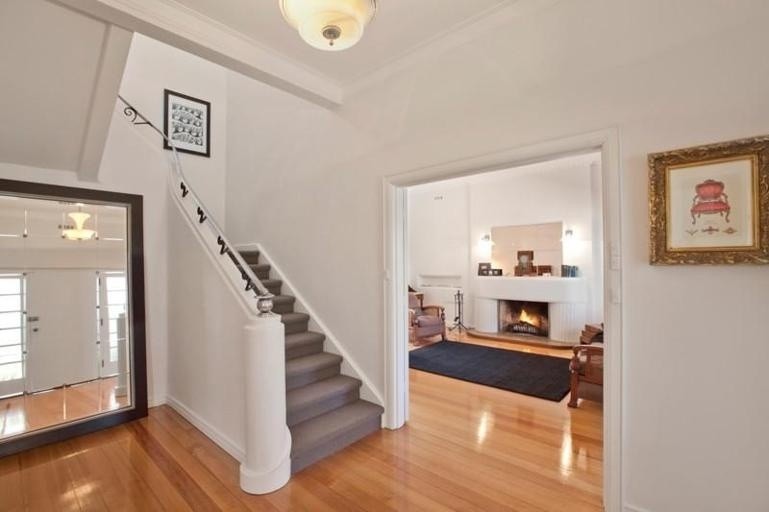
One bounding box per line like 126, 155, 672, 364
565, 230, 573, 240
62, 206, 99, 242
481, 235, 490, 242
278, 0, 377, 52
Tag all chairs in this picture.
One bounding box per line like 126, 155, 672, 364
408, 292, 446, 346
567, 336, 603, 408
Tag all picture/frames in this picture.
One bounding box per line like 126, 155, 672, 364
647, 135, 769, 266
163, 89, 211, 157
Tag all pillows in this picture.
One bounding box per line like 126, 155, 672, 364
409, 292, 420, 314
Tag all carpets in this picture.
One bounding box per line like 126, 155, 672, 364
409, 340, 572, 402
